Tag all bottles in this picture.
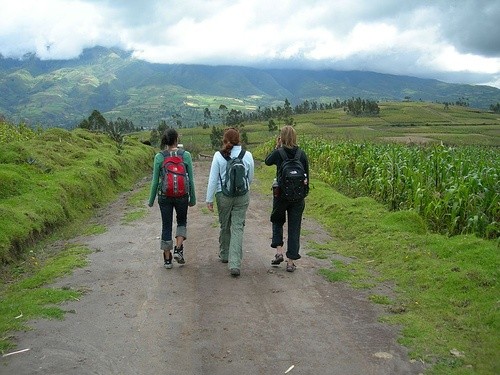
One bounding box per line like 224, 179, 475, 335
304, 173, 308, 195
273, 178, 279, 199
186, 172, 190, 193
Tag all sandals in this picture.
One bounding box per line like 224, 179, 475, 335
287, 263, 296, 271
271, 254, 284, 265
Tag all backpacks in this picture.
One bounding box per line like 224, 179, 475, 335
278, 148, 309, 201
159, 149, 189, 198
219, 148, 248, 197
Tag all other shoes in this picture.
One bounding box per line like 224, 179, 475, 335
231, 268, 240, 276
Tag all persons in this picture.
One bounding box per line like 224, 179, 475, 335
264, 126, 310, 272
148, 129, 196, 269
206, 127, 254, 276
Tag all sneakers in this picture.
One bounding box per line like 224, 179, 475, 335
173, 244, 185, 264
163, 252, 173, 269
